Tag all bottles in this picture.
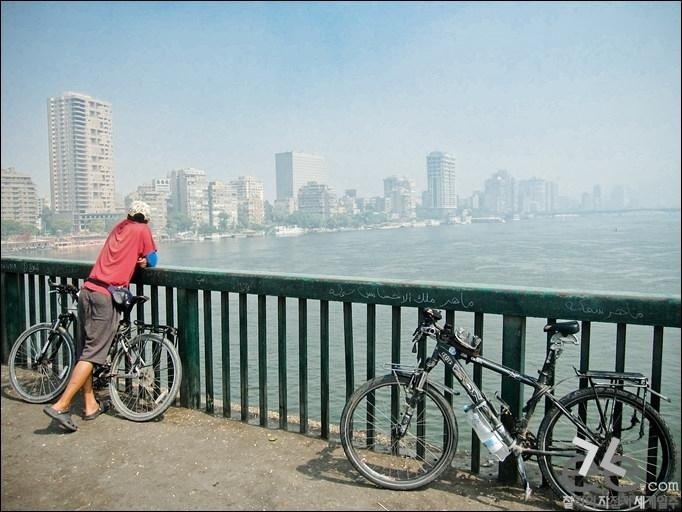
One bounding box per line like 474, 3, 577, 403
464, 403, 510, 462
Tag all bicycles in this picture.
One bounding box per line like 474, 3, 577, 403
7, 277, 185, 423
338, 306, 677, 511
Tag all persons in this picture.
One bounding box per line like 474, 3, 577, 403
42, 200, 159, 433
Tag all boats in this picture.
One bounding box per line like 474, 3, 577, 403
0, 224, 306, 256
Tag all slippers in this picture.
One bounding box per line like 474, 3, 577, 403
42, 405, 79, 432
84, 400, 112, 421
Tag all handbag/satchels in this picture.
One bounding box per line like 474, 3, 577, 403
85, 276, 133, 312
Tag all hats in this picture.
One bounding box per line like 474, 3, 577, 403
127, 200, 151, 221
